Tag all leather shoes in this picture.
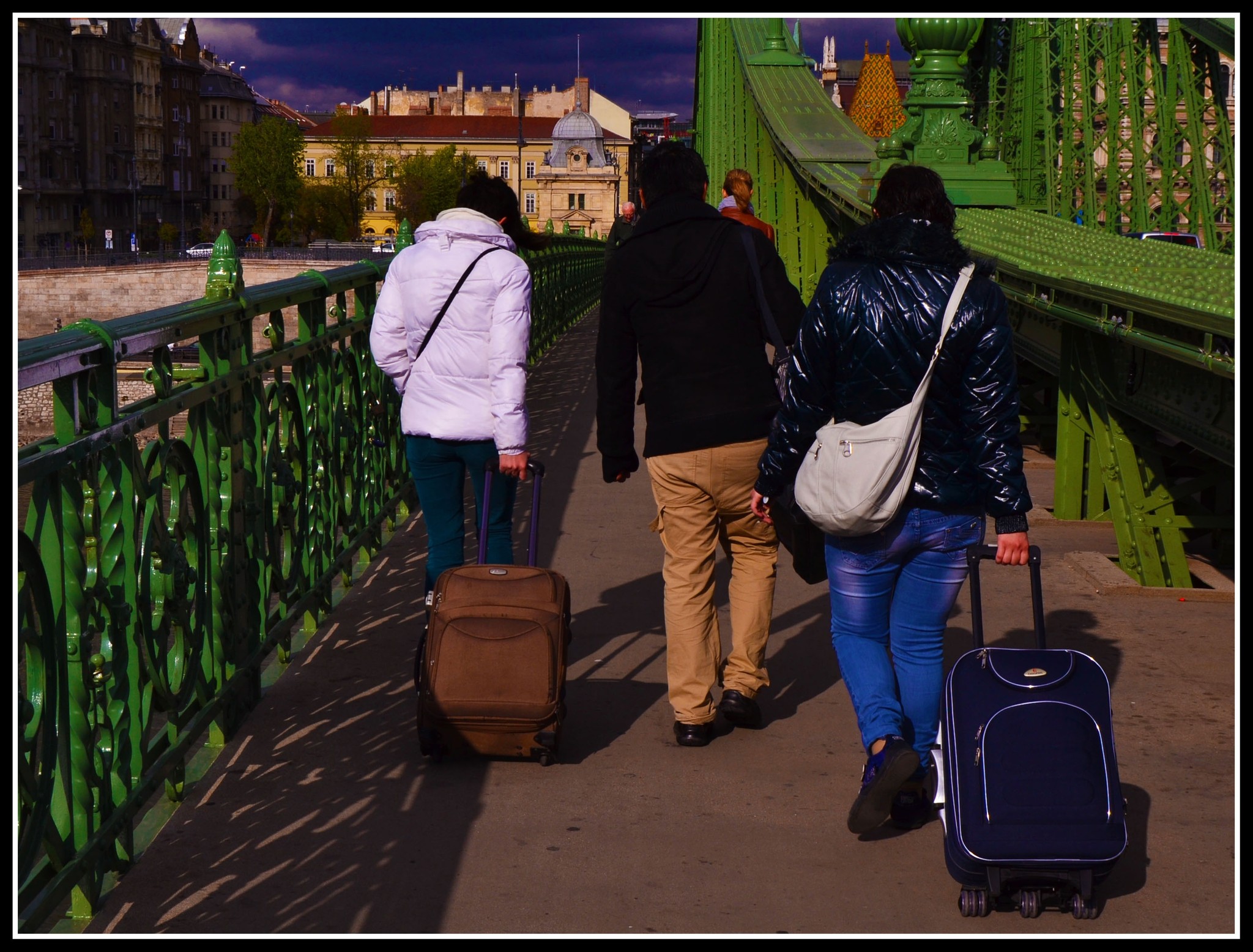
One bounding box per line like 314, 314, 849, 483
718, 689, 762, 728
673, 721, 713, 745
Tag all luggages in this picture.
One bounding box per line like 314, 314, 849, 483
412, 456, 569, 767
944, 543, 1130, 919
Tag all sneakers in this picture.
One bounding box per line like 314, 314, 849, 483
848, 734, 919, 832
895, 789, 931, 828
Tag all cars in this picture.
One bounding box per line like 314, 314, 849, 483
180, 243, 214, 259
371, 243, 396, 252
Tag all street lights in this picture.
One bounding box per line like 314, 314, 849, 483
460, 153, 467, 187
290, 211, 294, 253
128, 180, 143, 265
611, 155, 620, 218
518, 98, 533, 217
177, 141, 187, 259
18, 185, 38, 264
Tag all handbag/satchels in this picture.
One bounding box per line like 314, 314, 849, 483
794, 400, 924, 539
771, 352, 791, 400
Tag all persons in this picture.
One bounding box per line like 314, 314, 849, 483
717, 169, 775, 247
751, 163, 1034, 834
604, 202, 641, 267
370, 166, 533, 687
594, 140, 808, 747
